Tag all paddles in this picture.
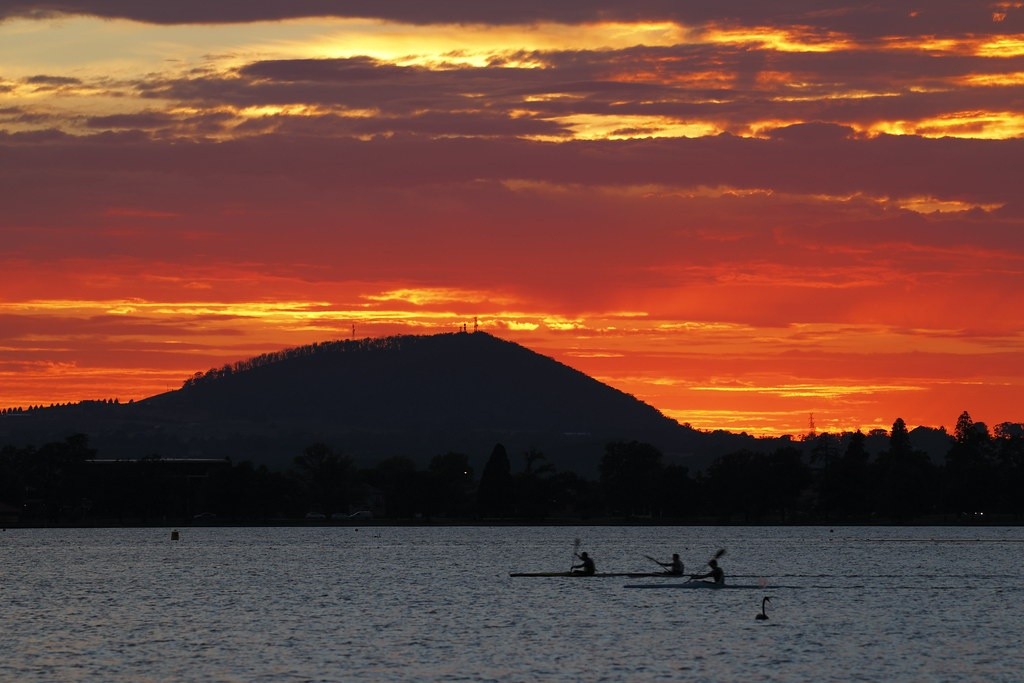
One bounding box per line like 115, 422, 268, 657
569, 539, 580, 572
642, 554, 670, 572
684, 548, 725, 583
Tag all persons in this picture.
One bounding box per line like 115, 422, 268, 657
661, 554, 683, 573
570, 552, 595, 574
693, 560, 724, 584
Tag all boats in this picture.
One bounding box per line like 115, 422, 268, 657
509, 568, 746, 578
622, 579, 804, 591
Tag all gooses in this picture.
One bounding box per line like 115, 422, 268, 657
755, 596, 771, 620
